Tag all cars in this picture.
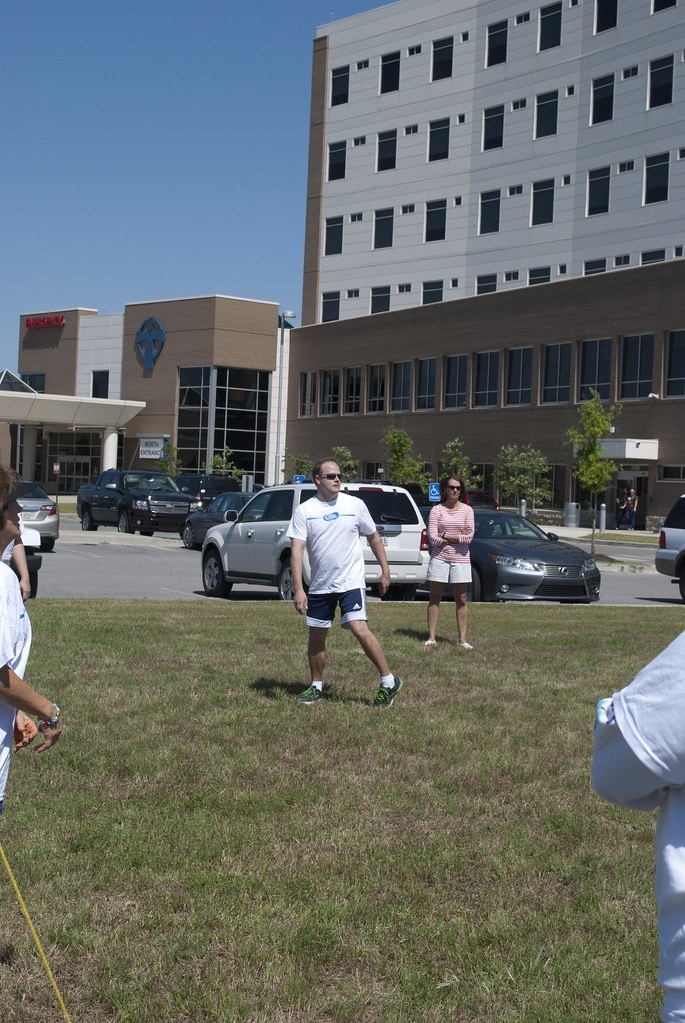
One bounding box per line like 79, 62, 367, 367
424, 490, 499, 509
655, 494, 685, 602
178, 491, 271, 550
419, 506, 601, 605
12, 480, 60, 552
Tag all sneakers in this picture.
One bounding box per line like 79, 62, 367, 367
372, 674, 403, 708
295, 685, 325, 706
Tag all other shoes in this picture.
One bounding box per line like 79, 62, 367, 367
457, 642, 473, 650
423, 640, 437, 646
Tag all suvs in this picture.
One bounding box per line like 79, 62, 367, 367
173, 473, 241, 507
201, 480, 430, 601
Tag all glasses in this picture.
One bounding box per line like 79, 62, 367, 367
446, 485, 462, 491
319, 473, 343, 480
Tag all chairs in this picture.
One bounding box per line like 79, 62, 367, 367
473, 525, 495, 538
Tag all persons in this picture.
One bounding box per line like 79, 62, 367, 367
590, 549, 685, 1023
615, 487, 639, 531
286, 456, 403, 711
422, 475, 475, 650
0, 466, 64, 816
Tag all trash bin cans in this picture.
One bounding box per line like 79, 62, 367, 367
563, 502, 581, 528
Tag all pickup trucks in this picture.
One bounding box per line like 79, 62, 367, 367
77, 468, 203, 540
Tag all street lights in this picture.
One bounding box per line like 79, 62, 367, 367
274, 311, 297, 485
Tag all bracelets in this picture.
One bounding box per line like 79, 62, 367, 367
37, 703, 60, 725
442, 532, 446, 539
